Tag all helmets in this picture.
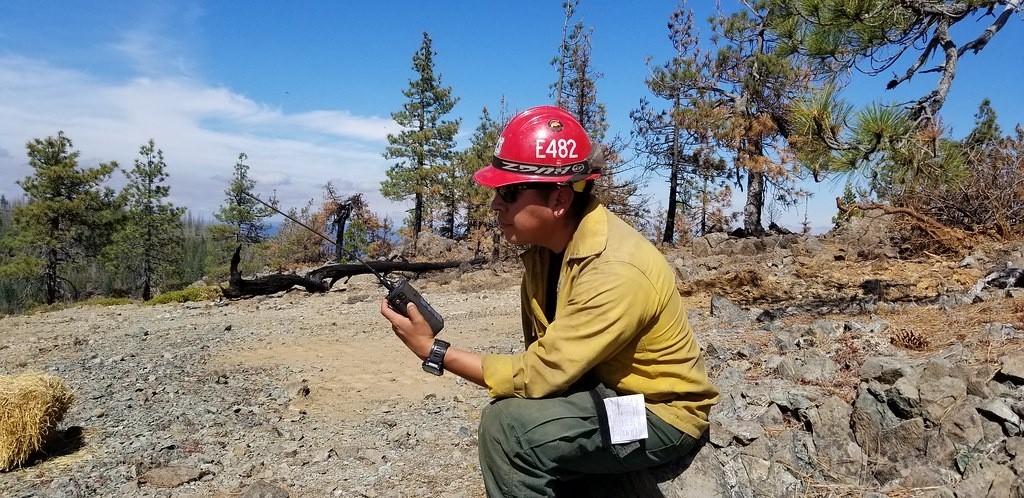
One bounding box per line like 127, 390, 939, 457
473, 105, 602, 188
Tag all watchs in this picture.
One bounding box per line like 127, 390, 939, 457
422, 338, 450, 376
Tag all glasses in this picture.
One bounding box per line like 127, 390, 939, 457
496, 183, 561, 204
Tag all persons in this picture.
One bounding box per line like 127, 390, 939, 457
380, 107, 721, 498
